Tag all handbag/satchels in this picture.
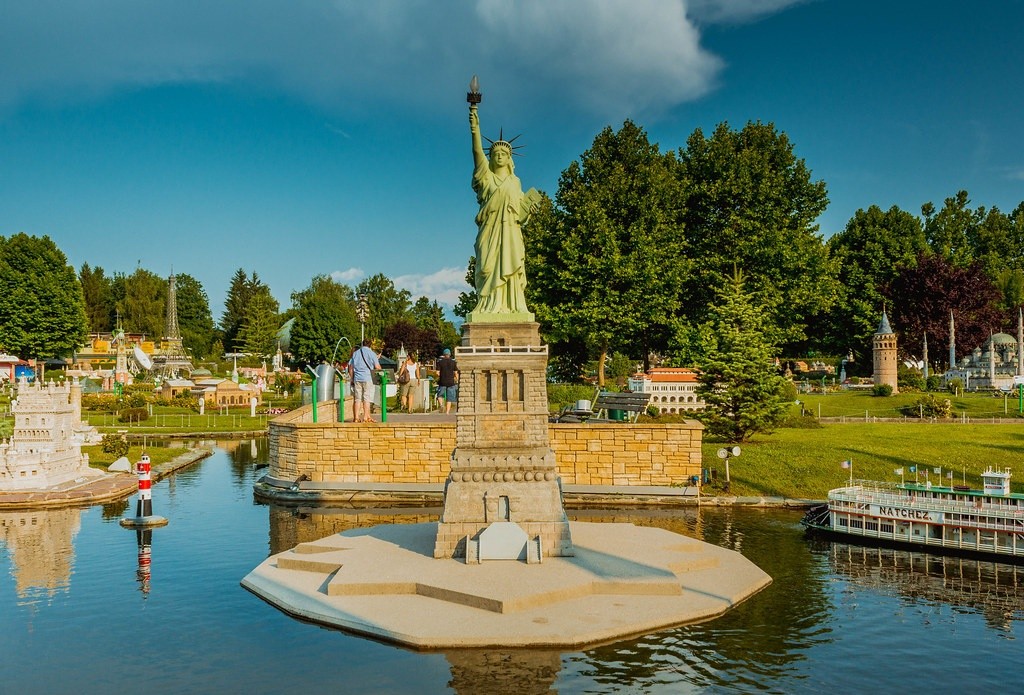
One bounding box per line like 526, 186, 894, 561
371, 369, 380, 385
398, 369, 411, 385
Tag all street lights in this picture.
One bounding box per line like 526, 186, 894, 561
355, 293, 370, 346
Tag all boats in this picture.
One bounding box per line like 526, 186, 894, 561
799, 463, 1024, 558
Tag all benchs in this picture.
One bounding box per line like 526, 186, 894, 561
560, 390, 651, 423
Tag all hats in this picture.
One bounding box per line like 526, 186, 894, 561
443, 349, 451, 355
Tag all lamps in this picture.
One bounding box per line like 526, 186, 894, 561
289, 474, 312, 493
722, 481, 730, 493
717, 445, 741, 459
253, 463, 270, 472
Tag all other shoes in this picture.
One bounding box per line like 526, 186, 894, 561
400, 405, 406, 413
362, 418, 378, 424
408, 409, 413, 414
353, 418, 362, 423
439, 405, 444, 413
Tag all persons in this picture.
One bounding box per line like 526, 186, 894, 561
348, 339, 381, 423
468, 112, 540, 313
399, 351, 420, 413
436, 349, 458, 413
